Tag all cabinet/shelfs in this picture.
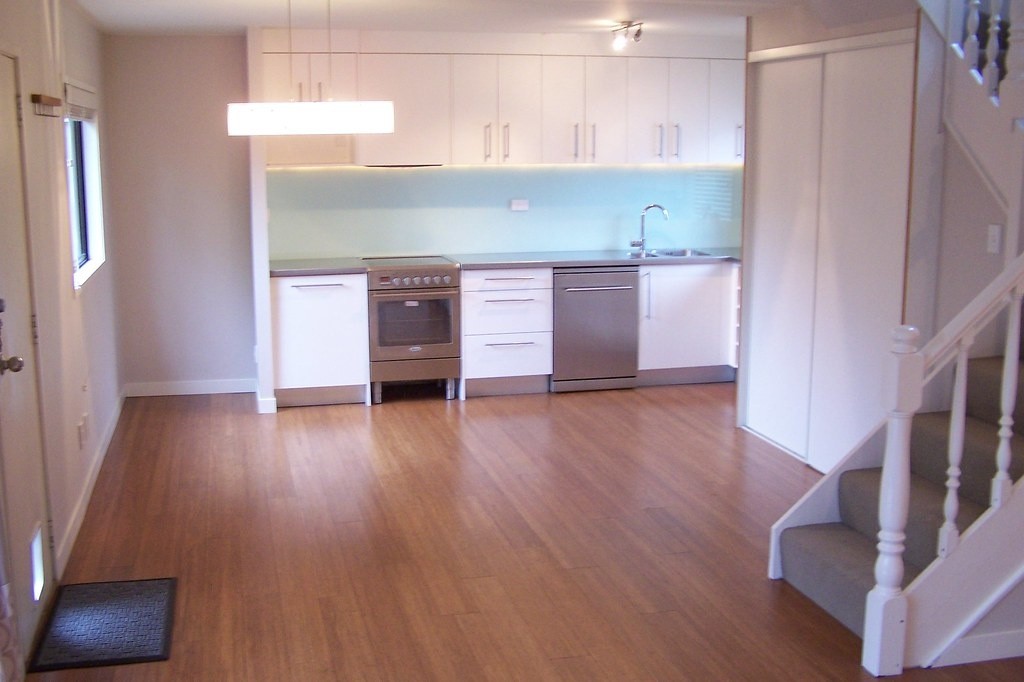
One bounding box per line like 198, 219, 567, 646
736, 10, 945, 475
270, 274, 370, 389
459, 268, 554, 379
638, 263, 739, 371
261, 27, 744, 165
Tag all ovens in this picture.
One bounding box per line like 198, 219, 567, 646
368, 290, 460, 383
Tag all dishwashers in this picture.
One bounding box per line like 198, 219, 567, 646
549, 272, 638, 393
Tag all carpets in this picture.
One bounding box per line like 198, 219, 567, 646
25, 576, 176, 673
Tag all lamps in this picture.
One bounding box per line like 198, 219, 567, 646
612, 20, 643, 51
225, 0, 395, 138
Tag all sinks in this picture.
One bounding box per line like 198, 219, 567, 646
648, 247, 711, 258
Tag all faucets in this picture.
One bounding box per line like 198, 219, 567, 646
630, 203, 668, 255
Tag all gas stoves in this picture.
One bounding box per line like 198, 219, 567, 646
362, 255, 462, 290
